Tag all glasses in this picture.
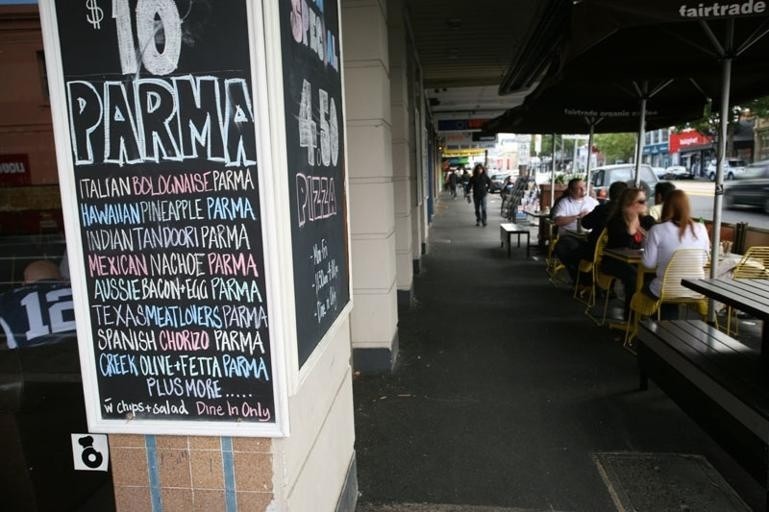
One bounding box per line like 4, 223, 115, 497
634, 199, 646, 205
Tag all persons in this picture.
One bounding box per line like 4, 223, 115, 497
597, 187, 655, 323
0, 260, 76, 350
461, 170, 472, 203
549, 177, 676, 298
449, 171, 458, 198
640, 190, 710, 321
465, 164, 490, 226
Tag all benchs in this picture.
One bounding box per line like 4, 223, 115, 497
638, 316, 769, 490
501, 222, 529, 259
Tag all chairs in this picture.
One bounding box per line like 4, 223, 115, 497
544, 227, 620, 326
627, 248, 716, 352
718, 245, 769, 339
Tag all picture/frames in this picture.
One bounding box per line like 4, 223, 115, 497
263, 0, 355, 395
39, 0, 292, 441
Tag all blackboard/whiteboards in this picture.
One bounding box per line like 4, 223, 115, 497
279, 1, 349, 371
52, 0, 275, 421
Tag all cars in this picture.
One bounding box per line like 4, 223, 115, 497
487, 174, 505, 194
502, 174, 539, 200
664, 165, 695, 181
653, 167, 666, 178
723, 159, 769, 214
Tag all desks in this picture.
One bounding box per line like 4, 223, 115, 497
522, 208, 550, 260
682, 275, 769, 324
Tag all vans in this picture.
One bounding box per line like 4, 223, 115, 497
585, 164, 661, 210
705, 157, 746, 181
